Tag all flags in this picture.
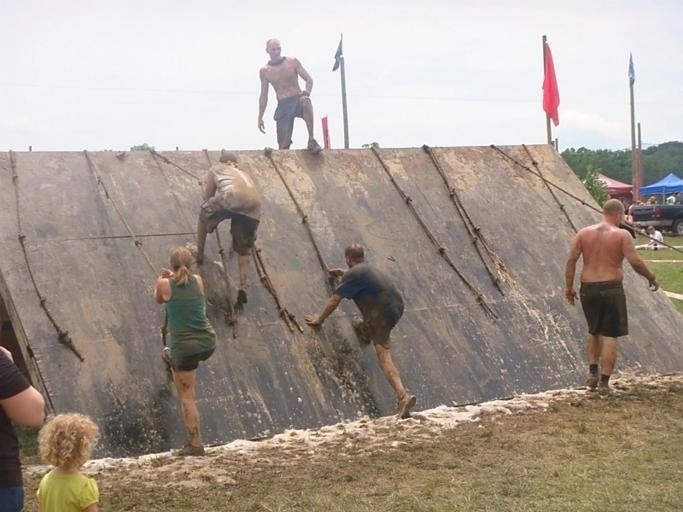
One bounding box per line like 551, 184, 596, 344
333, 40, 341, 73
542, 43, 560, 125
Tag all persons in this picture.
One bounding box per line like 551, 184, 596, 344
646, 197, 659, 205
1, 347, 47, 511
35, 414, 101, 512
646, 224, 663, 251
564, 198, 659, 399
258, 38, 322, 152
185, 152, 261, 304
306, 241, 417, 421
156, 247, 217, 456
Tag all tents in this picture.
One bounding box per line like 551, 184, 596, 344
582, 171, 633, 199
637, 173, 682, 205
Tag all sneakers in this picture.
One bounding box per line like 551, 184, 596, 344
162, 347, 172, 363
238, 287, 248, 304
179, 445, 205, 456
587, 375, 599, 388
597, 383, 612, 396
186, 242, 204, 262
309, 140, 321, 153
399, 394, 416, 420
353, 316, 371, 344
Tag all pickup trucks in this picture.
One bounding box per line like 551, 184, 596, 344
628, 205, 682, 236
